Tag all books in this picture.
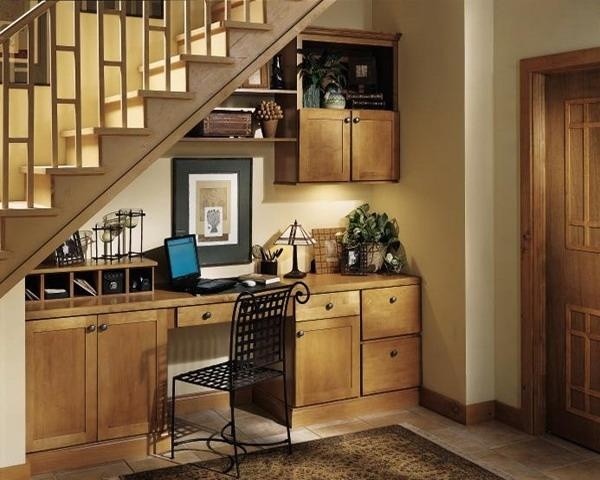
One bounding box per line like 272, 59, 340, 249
24, 287, 40, 300
70, 277, 98, 298
238, 271, 280, 286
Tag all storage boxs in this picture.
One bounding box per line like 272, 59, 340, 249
184, 106, 255, 138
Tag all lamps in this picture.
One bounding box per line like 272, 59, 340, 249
273, 220, 319, 279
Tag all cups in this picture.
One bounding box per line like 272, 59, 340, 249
261, 260, 278, 275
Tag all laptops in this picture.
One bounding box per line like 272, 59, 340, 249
164, 235, 236, 294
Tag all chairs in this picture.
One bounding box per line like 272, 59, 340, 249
170, 281, 311, 479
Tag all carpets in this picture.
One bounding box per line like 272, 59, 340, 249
101, 424, 505, 480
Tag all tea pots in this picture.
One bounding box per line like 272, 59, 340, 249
322, 87, 346, 110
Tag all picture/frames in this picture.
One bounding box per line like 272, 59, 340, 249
242, 61, 269, 89
0, 0, 51, 87
171, 157, 254, 268
80, 0, 164, 20
311, 227, 347, 275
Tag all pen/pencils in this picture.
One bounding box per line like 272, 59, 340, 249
260, 248, 283, 261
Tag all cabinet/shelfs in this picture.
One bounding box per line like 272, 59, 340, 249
273, 23, 404, 187
253, 268, 424, 429
25, 255, 159, 311
179, 88, 298, 141
26, 273, 304, 476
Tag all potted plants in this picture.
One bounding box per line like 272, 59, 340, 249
295, 44, 353, 109
334, 200, 402, 273
253, 100, 285, 138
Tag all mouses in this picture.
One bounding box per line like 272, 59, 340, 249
242, 279, 256, 288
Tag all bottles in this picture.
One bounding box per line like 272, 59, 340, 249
271, 54, 287, 89
